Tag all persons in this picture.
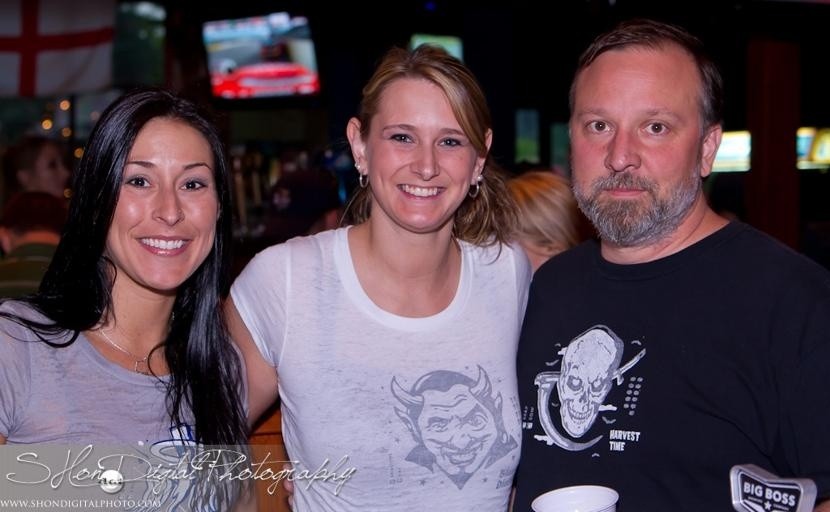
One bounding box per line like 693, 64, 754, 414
2, 135, 71, 198
259, 30, 294, 63
0, 191, 68, 299
282, 18, 830, 512
0, 88, 256, 512
223, 42, 533, 512
504, 171, 602, 281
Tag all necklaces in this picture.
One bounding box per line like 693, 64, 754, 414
96, 324, 165, 371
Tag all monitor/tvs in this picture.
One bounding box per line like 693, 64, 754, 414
182, 1, 331, 119
707, 126, 752, 174
795, 123, 830, 171
404, 30, 463, 64
